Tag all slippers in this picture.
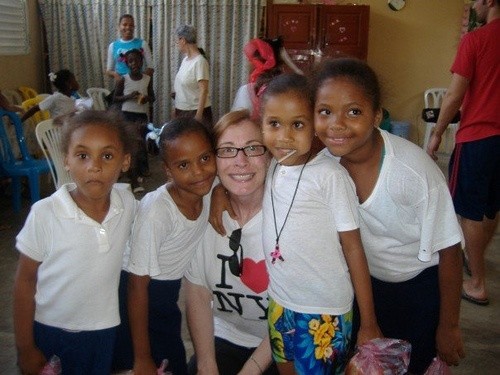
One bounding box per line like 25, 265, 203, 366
462, 281, 489, 305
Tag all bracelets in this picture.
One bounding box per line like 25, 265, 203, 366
249, 356, 263, 374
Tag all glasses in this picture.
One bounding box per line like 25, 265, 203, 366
227, 228, 244, 277
215, 145, 267, 158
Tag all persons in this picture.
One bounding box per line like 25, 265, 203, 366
14, 109, 142, 375
186, 109, 278, 375
426, 0, 500, 305
113, 48, 155, 123
170, 26, 214, 130
230, 39, 285, 121
11, 68, 79, 126
106, 15, 154, 89
0, 92, 24, 113
314, 59, 467, 375
209, 73, 385, 375
110, 118, 219, 375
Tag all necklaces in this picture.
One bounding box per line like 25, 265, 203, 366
270, 150, 313, 263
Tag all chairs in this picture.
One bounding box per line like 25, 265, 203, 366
0, 85, 111, 216
422, 87, 457, 154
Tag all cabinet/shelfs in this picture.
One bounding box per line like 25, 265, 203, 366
262, 4, 371, 77
